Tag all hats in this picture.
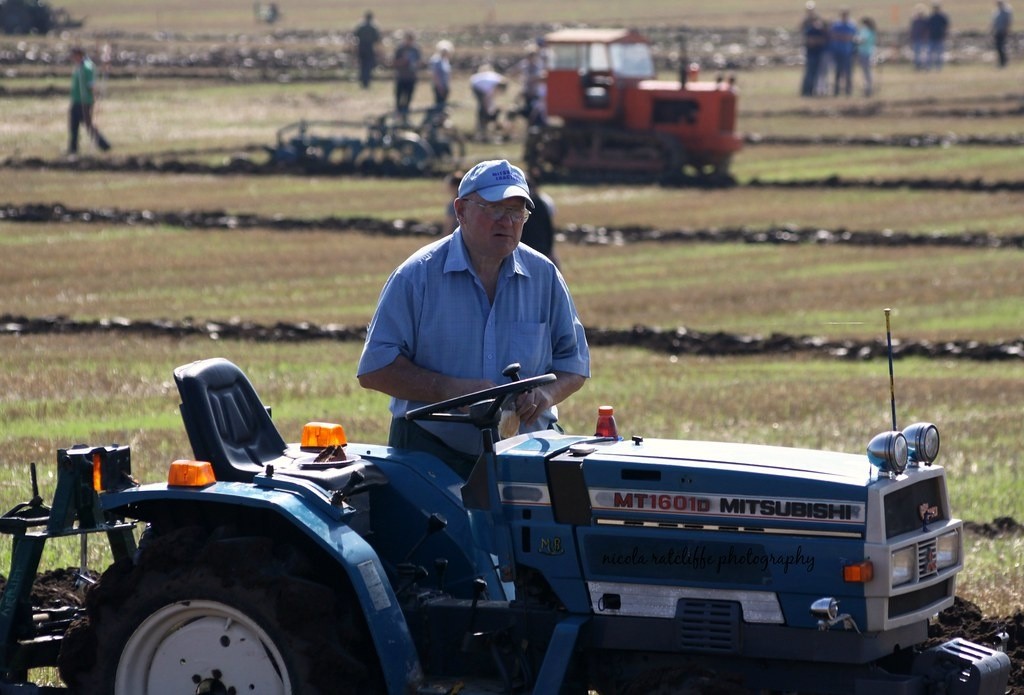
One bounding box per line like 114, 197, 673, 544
458, 159, 535, 208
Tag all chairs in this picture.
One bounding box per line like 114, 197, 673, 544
172, 355, 371, 501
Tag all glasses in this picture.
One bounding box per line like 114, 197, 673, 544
463, 198, 531, 224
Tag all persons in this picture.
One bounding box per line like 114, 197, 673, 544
392, 31, 422, 118
430, 42, 454, 126
801, 3, 876, 97
519, 37, 548, 124
64, 44, 112, 154
358, 160, 590, 482
354, 11, 381, 88
991, 0, 1013, 68
909, 4, 950, 69
470, 63, 511, 140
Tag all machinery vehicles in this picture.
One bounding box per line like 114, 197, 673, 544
0, 307, 1015, 695
521, 27, 744, 188
